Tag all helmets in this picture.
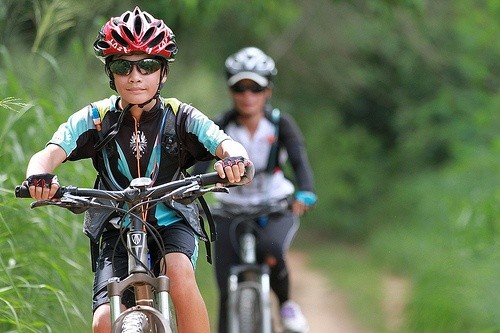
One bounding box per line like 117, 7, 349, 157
93, 5, 178, 65
225, 47, 280, 90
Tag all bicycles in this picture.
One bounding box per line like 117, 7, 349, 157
15, 166, 247, 333
197, 197, 313, 333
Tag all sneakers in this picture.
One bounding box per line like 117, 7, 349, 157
280, 297, 308, 332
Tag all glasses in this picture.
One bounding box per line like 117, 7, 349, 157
105, 57, 165, 77
230, 83, 263, 92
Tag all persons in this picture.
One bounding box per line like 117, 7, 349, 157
26, 6, 247, 333
189, 45, 317, 333
